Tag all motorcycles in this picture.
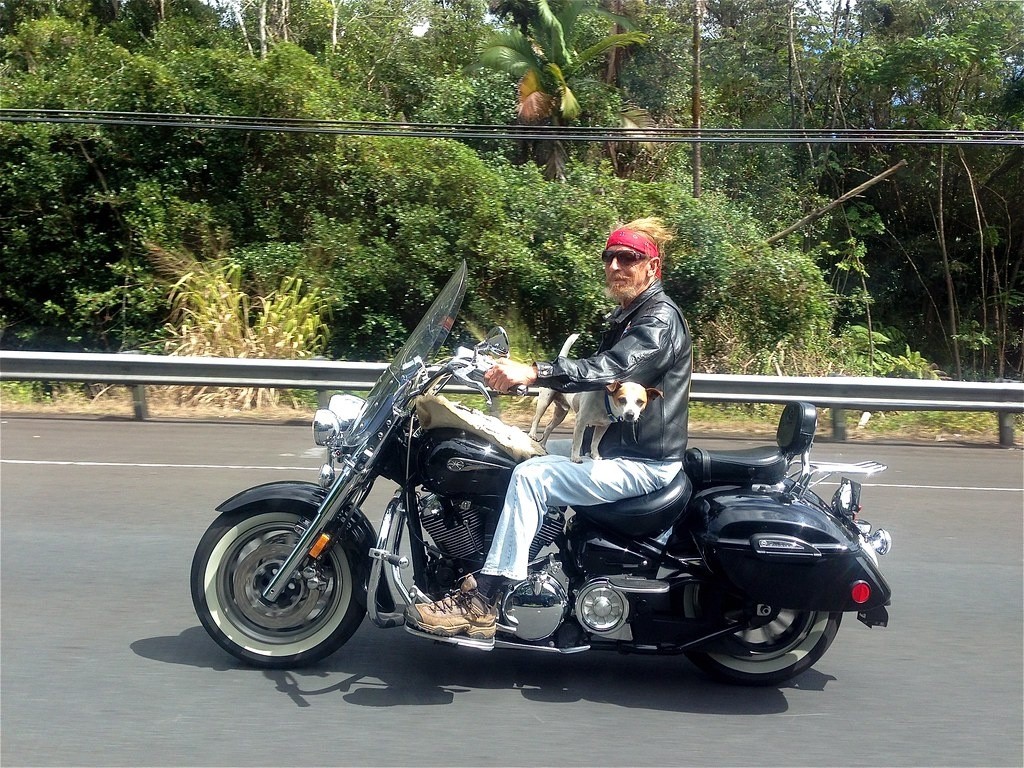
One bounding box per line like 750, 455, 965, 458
191, 258, 891, 687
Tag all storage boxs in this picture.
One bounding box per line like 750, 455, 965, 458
698, 503, 892, 613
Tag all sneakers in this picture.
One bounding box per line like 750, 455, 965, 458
403, 581, 498, 639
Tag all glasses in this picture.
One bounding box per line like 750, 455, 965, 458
602, 250, 646, 266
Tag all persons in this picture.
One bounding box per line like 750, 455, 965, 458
404, 218, 691, 641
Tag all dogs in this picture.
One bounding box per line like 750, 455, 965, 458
528, 333, 664, 463
